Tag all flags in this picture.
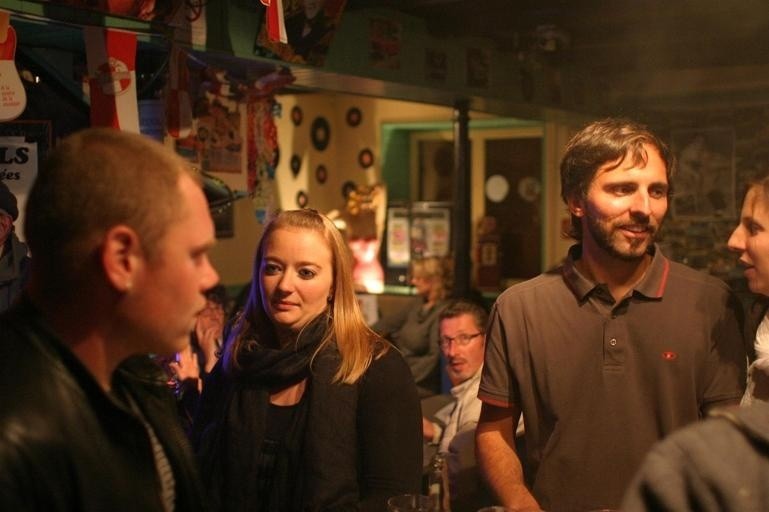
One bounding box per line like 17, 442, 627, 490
82, 29, 142, 136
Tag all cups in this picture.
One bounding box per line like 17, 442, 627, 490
438, 332, 484, 348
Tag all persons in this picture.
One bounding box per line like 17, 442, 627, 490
255, 1, 334, 63
475, 118, 744, 510
623, 403, 767, 509
372, 256, 454, 387
422, 301, 525, 509
190, 209, 420, 509
725, 171, 767, 405
2, 131, 225, 511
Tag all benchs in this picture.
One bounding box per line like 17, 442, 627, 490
82, 29, 142, 136
